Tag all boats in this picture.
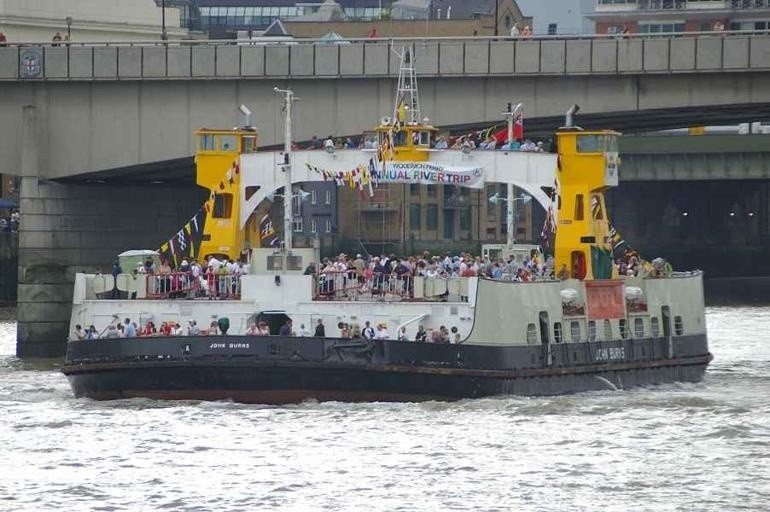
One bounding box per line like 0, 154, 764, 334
60, 47, 716, 402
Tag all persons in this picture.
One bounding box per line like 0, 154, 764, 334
82, 255, 249, 300
303, 250, 569, 302
511, 23, 519, 41
52, 32, 62, 46
291, 130, 544, 152
714, 20, 724, 37
0, 32, 7, 47
368, 29, 380, 43
521, 26, 532, 41
615, 249, 673, 277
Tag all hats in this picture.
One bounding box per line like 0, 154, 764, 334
181, 260, 189, 265
